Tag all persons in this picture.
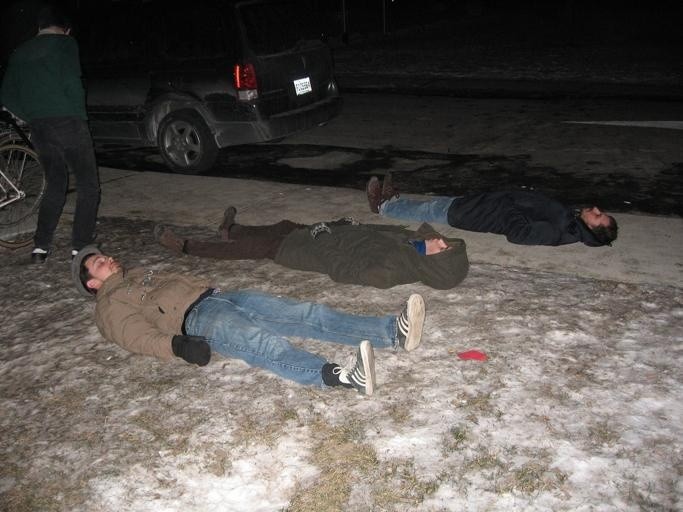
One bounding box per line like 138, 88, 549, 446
1, 11, 101, 263
366, 170, 617, 247
153, 205, 470, 289
70, 244, 425, 394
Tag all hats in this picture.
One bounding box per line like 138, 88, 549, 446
69, 246, 104, 297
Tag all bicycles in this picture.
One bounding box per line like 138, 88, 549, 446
0, 112, 50, 249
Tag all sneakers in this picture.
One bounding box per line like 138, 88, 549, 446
30, 248, 54, 267
153, 224, 186, 253
216, 204, 237, 238
395, 291, 426, 352
381, 172, 401, 201
365, 175, 383, 214
331, 339, 378, 397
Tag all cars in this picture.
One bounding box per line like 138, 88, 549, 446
75, 7, 344, 174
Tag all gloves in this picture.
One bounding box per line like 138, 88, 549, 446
170, 334, 214, 367
